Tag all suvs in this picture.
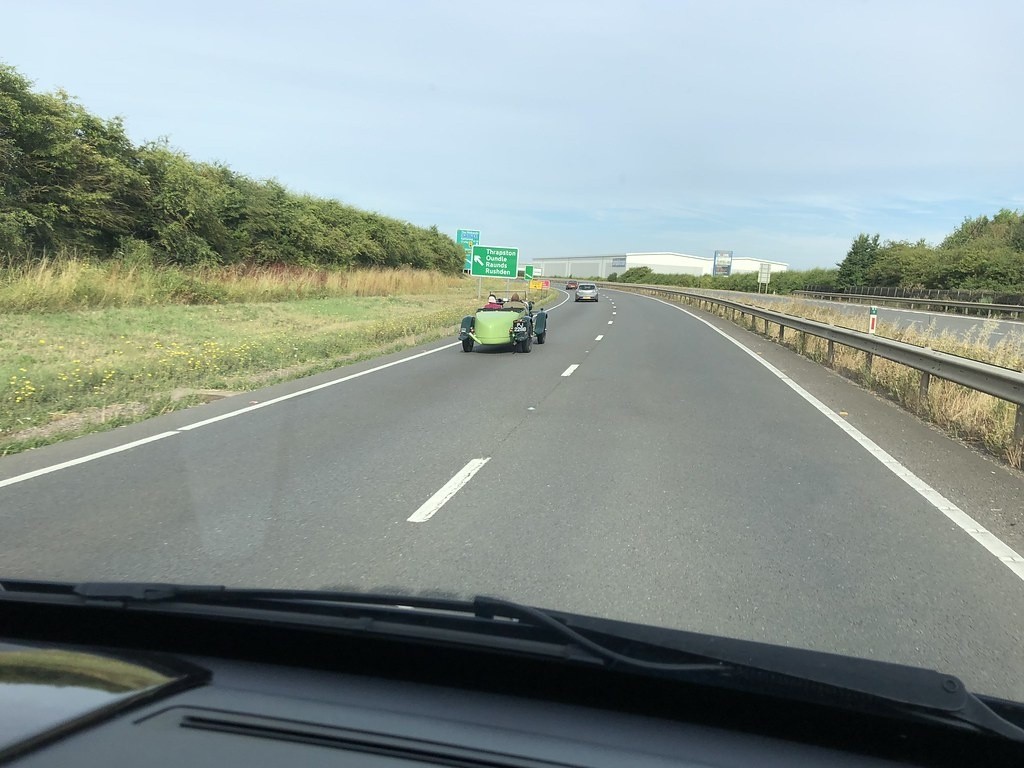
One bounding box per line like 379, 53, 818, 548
566, 281, 578, 290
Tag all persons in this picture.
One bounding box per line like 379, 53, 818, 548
485, 295, 502, 308
511, 293, 525, 304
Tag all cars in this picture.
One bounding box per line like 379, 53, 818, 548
575, 284, 600, 302
458, 289, 547, 353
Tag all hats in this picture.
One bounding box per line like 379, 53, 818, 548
512, 294, 519, 300
488, 296, 496, 303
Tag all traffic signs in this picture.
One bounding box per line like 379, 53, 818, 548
471, 246, 518, 280
